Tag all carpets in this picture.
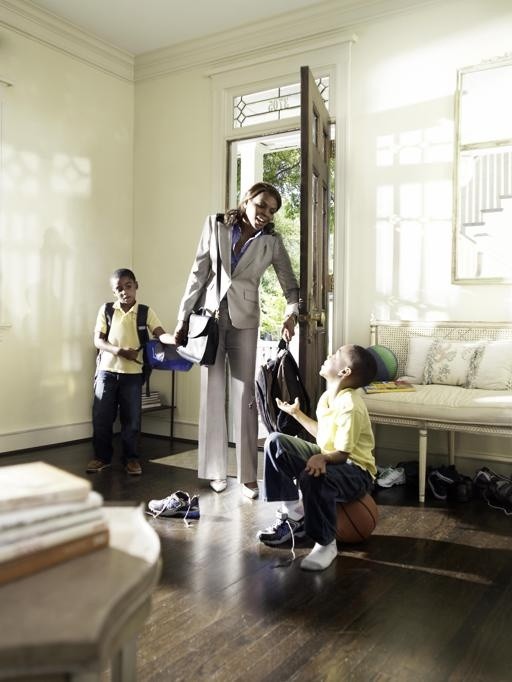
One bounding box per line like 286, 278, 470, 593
148, 446, 265, 482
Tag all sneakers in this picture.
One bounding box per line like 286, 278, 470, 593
375, 464, 407, 488
427, 460, 474, 503
126, 461, 142, 475
257, 509, 305, 546
149, 489, 202, 519
86, 459, 113, 473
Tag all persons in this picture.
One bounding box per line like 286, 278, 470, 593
173, 181, 300, 498
86, 268, 188, 476
254, 342, 379, 572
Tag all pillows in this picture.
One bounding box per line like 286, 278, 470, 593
396, 336, 512, 391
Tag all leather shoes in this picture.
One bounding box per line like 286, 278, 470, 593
210, 480, 228, 492
243, 485, 260, 500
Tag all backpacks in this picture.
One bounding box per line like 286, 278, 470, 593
255, 337, 312, 438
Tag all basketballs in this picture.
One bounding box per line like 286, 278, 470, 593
366, 345, 397, 381
334, 492, 380, 543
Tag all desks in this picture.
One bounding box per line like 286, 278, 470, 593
0, 501, 163, 681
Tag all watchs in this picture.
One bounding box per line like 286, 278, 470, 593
289, 316, 296, 325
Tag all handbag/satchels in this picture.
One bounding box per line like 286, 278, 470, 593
146, 339, 193, 372
174, 305, 220, 367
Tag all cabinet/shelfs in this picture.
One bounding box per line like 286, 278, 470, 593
137, 369, 177, 447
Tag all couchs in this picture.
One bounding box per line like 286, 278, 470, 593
355, 314, 512, 504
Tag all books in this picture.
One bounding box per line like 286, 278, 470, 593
362, 380, 418, 395
